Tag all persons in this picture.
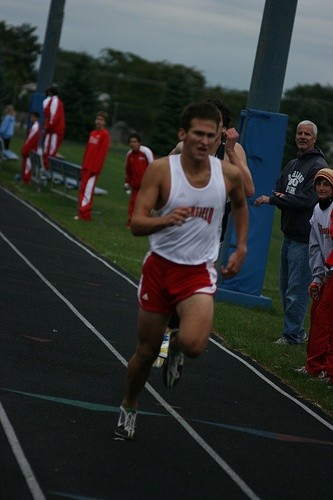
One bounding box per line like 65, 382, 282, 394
110, 103, 248, 442
0, 87, 333, 375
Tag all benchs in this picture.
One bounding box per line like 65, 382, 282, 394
0, 135, 108, 215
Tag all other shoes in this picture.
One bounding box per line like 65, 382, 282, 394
273, 334, 308, 345
319, 372, 333, 384
294, 366, 309, 374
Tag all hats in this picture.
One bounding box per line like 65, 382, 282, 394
313, 168, 333, 189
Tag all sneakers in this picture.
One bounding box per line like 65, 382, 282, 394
114, 405, 137, 441
161, 329, 184, 389
